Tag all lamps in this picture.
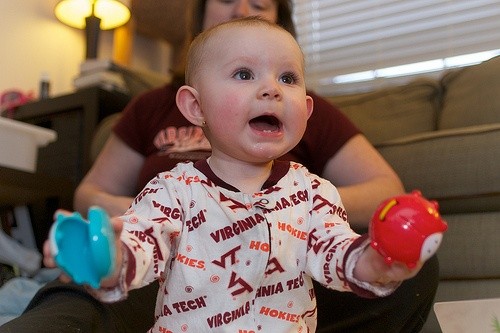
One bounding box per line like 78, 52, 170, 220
54, 0, 135, 61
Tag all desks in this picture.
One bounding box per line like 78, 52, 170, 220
0, 115, 57, 173
0, 84, 134, 232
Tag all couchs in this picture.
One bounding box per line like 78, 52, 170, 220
322, 54, 500, 281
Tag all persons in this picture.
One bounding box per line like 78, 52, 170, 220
0, 0, 442, 333
43, 15, 425, 333
